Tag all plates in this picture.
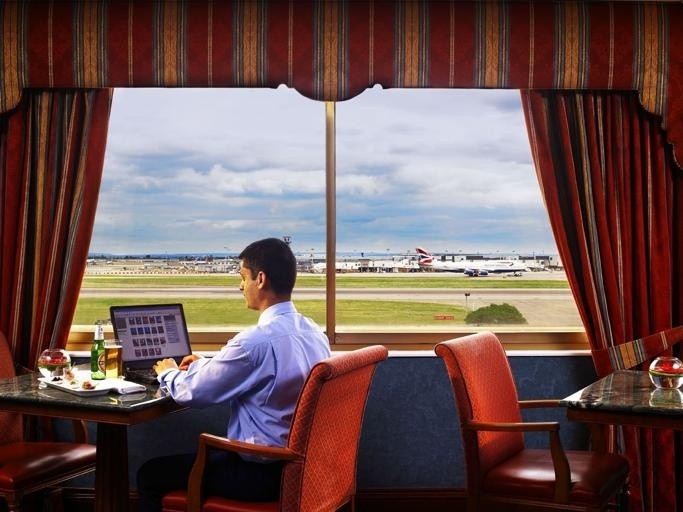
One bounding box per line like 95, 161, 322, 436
37, 375, 114, 398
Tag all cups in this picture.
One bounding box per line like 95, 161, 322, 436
103, 338, 123, 382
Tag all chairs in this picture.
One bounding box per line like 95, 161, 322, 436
1, 335, 97, 511
435, 333, 605, 512
164, 344, 391, 510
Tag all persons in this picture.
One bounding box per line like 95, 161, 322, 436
136, 238, 331, 512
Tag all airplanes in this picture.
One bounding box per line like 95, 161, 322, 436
415, 247, 531, 277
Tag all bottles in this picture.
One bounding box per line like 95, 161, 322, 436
90, 318, 106, 382
649, 356, 683, 390
36, 347, 72, 379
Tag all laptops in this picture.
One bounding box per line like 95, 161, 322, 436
106, 304, 193, 383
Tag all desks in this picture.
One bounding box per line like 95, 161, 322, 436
560, 367, 682, 510
3, 360, 192, 510
